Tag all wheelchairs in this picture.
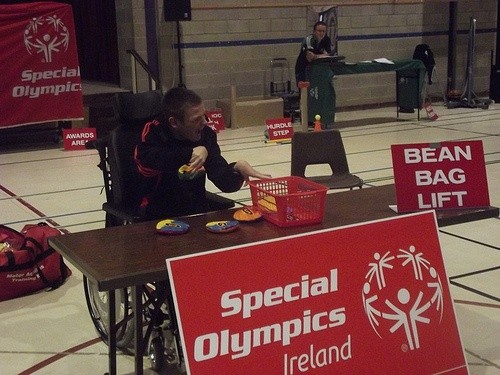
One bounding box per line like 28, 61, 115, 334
84, 88, 235, 375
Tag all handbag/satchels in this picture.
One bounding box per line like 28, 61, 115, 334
0, 222, 72, 301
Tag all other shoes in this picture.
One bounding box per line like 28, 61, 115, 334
300, 118, 315, 128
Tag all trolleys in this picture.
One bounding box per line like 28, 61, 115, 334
271, 57, 300, 123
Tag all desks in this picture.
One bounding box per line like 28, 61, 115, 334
46, 183, 500, 375
307, 59, 426, 128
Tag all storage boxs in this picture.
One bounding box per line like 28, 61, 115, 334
216, 95, 284, 129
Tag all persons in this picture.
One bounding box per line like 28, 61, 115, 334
295, 21, 333, 131
126, 87, 272, 225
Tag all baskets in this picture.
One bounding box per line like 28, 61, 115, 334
248, 176, 329, 228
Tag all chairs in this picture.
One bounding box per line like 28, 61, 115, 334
291, 129, 363, 191
268, 58, 301, 124
